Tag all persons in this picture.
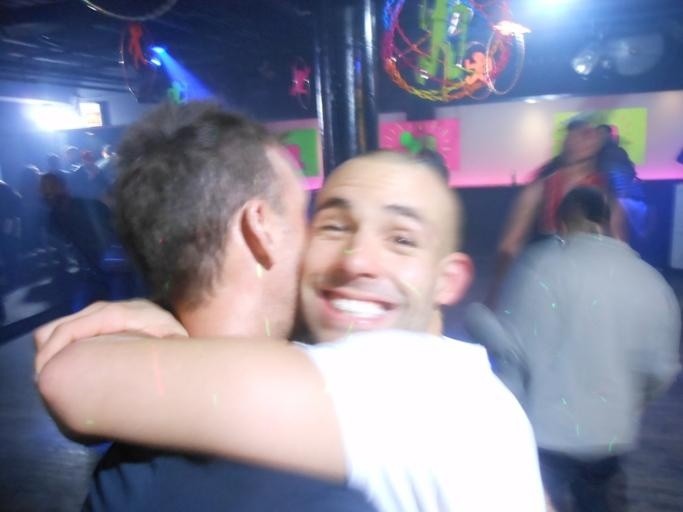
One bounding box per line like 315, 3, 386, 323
482, 183, 682, 507
75, 105, 376, 507
35, 149, 552, 507
0, 121, 156, 305
499, 115, 652, 262
